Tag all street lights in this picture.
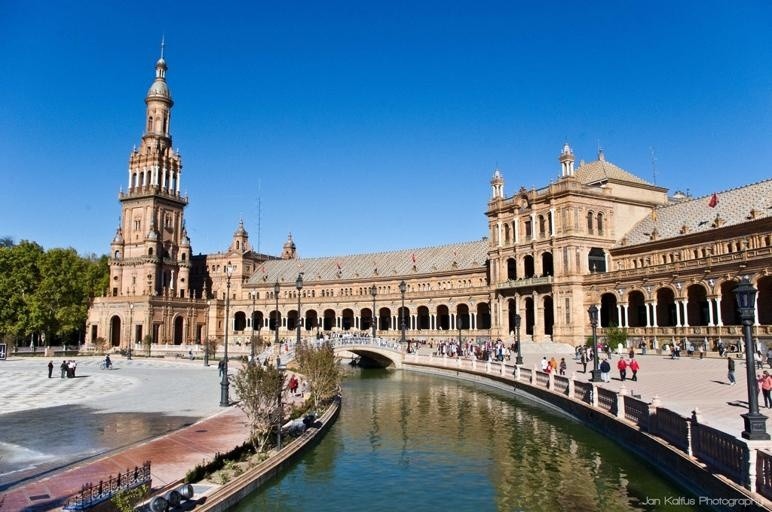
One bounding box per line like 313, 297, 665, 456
734, 273, 770, 438
372, 283, 377, 337
274, 280, 281, 373
514, 313, 523, 364
294, 275, 304, 345
587, 303, 600, 380
129, 302, 134, 352
454, 314, 463, 351
219, 260, 236, 394
399, 280, 405, 340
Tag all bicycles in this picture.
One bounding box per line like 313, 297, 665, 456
99, 362, 112, 370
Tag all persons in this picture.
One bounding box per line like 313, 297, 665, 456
288, 374, 299, 393
670, 341, 738, 359
600, 358, 611, 383
575, 345, 594, 373
105, 353, 112, 368
61, 359, 77, 378
436, 337, 511, 362
757, 350, 764, 370
766, 348, 772, 369
217, 358, 224, 376
757, 370, 772, 409
316, 330, 336, 346
48, 360, 54, 378
618, 346, 640, 381
255, 354, 280, 373
541, 357, 566, 375
727, 357, 736, 386
